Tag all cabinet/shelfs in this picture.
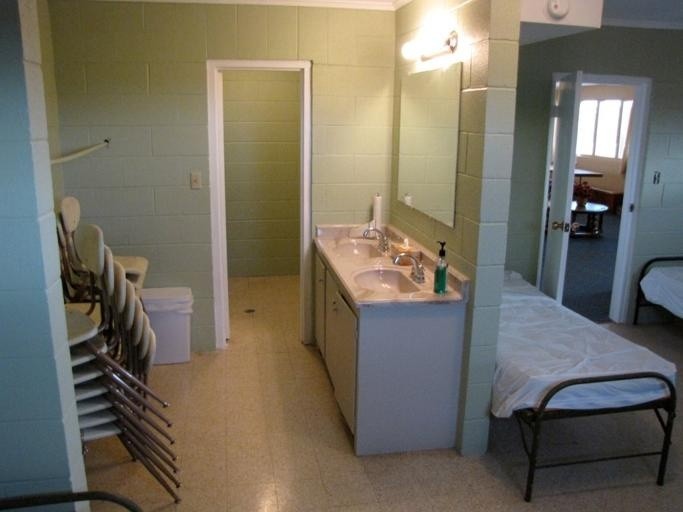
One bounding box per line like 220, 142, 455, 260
313, 247, 467, 457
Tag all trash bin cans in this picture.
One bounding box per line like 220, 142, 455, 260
142, 288, 194, 365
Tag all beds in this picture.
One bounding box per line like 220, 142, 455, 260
632, 256, 683, 325
490, 271, 677, 502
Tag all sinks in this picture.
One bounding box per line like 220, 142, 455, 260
354, 269, 421, 293
332, 244, 383, 259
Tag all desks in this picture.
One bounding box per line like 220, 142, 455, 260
571, 200, 610, 239
574, 167, 604, 194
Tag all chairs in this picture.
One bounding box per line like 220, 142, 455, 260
55, 195, 182, 504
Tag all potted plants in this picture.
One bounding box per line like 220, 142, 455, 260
574, 181, 593, 205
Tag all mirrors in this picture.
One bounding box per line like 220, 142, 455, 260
396, 60, 464, 230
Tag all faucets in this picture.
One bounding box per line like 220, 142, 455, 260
363, 228, 389, 252
393, 253, 425, 284
